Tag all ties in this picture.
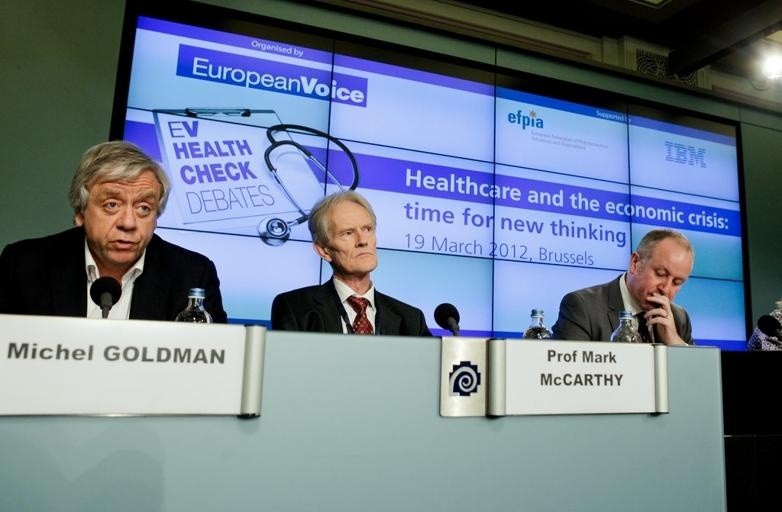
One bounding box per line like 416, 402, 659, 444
637, 311, 654, 342
346, 295, 374, 335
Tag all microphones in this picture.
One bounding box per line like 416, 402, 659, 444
89, 276, 122, 319
434, 303, 462, 335
757, 314, 782, 343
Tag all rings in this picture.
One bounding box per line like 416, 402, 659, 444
661, 310, 669, 319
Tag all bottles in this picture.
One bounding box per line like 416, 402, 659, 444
524, 308, 553, 339
175, 288, 214, 323
611, 311, 642, 343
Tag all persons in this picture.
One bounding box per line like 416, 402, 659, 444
270, 191, 434, 337
1, 140, 228, 323
551, 228, 696, 345
